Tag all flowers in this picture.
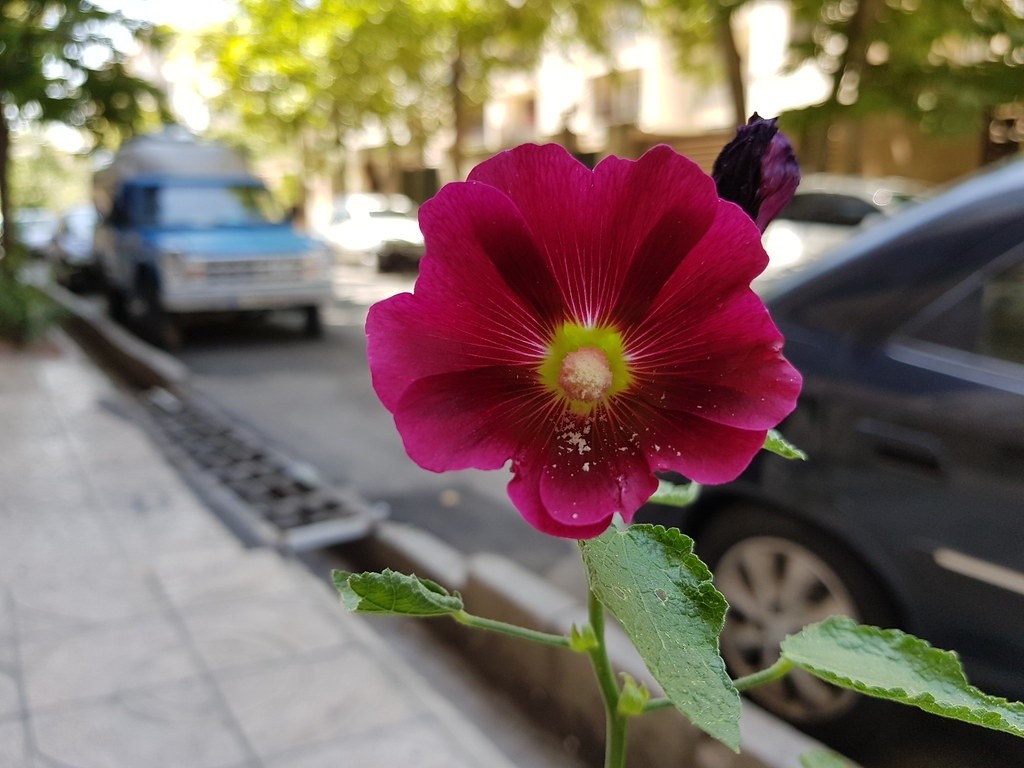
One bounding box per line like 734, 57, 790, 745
327, 140, 1024, 768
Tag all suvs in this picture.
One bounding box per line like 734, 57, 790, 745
91, 129, 335, 345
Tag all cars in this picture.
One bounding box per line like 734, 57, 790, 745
747, 174, 928, 295
631, 144, 1023, 745
13, 212, 52, 259
317, 191, 433, 270
46, 204, 104, 293
